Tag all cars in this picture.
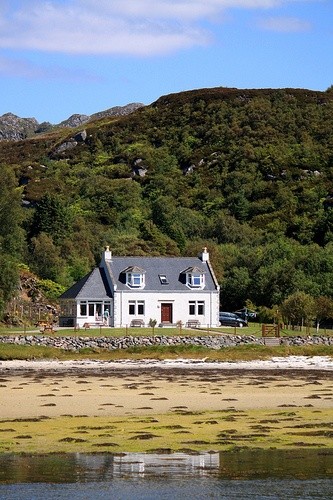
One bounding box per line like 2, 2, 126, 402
219, 312, 246, 327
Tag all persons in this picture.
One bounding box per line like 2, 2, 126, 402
103, 309, 109, 326
